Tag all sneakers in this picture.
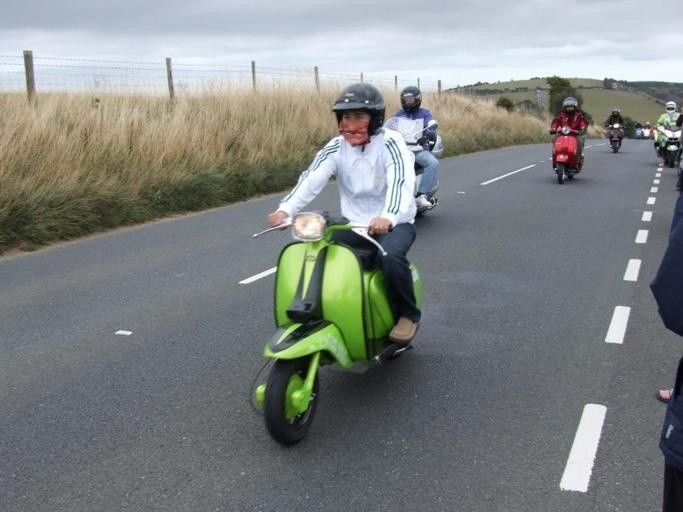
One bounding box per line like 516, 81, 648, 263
389, 316, 418, 343
414, 194, 432, 206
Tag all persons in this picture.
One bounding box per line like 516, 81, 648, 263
649, 113, 683, 511
267, 84, 422, 345
605, 100, 681, 145
551, 97, 588, 164
383, 86, 439, 206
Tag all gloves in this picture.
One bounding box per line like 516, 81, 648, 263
579, 128, 586, 135
549, 129, 556, 134
417, 136, 431, 146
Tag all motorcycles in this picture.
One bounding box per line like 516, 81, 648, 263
634, 128, 652, 139
250, 210, 423, 445
407, 120, 444, 216
605, 124, 624, 153
654, 130, 683, 168
550, 125, 584, 184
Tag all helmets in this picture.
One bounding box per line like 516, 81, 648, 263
612, 108, 620, 116
332, 84, 385, 111
563, 97, 577, 112
665, 101, 676, 114
401, 86, 421, 111
